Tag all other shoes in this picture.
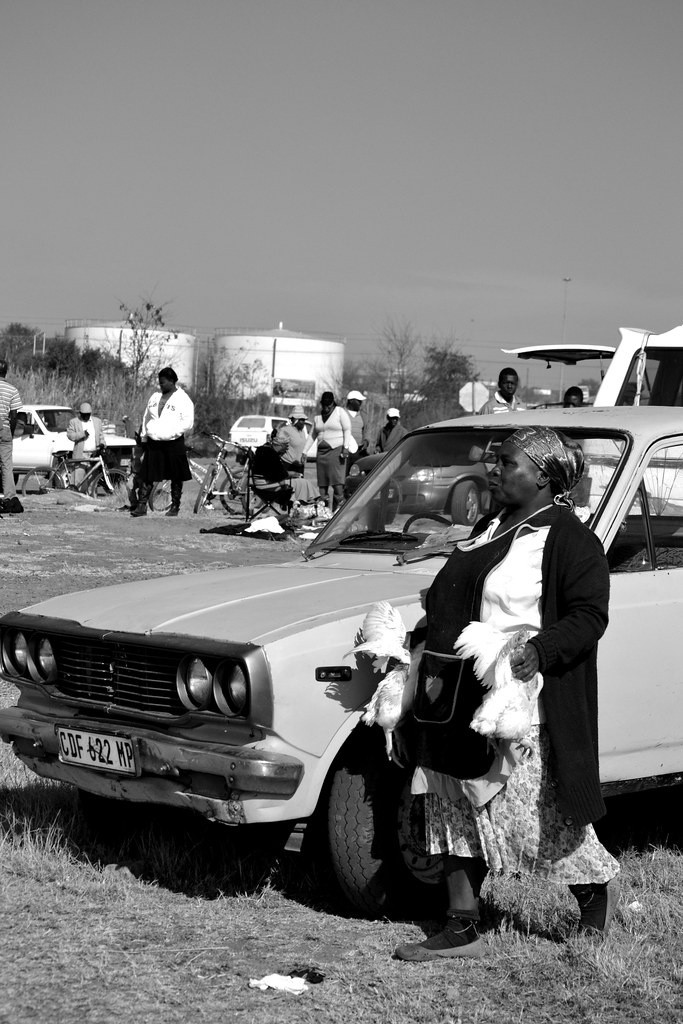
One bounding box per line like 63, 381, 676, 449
578, 878, 621, 944
394, 923, 483, 963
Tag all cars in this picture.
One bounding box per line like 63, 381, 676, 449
0, 402, 683, 926
340, 441, 496, 527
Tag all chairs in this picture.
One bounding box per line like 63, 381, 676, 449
246, 447, 304, 521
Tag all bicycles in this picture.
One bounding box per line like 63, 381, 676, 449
19, 444, 134, 512
147, 437, 283, 523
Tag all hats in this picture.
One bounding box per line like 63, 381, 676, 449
321, 391, 334, 406
347, 390, 367, 401
288, 406, 308, 420
387, 408, 401, 418
80, 403, 92, 414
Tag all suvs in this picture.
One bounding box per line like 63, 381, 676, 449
0, 405, 137, 493
227, 414, 320, 463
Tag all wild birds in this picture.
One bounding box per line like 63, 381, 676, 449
454, 621, 545, 759
340, 600, 423, 768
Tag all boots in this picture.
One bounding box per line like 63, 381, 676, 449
164, 479, 182, 516
131, 480, 153, 516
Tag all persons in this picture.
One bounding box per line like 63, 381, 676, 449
0, 360, 26, 513
344, 391, 371, 476
301, 392, 351, 510
391, 426, 622, 961
479, 367, 530, 414
375, 408, 410, 452
130, 367, 194, 517
563, 385, 583, 409
17, 411, 34, 434
250, 406, 308, 513
67, 402, 106, 493
